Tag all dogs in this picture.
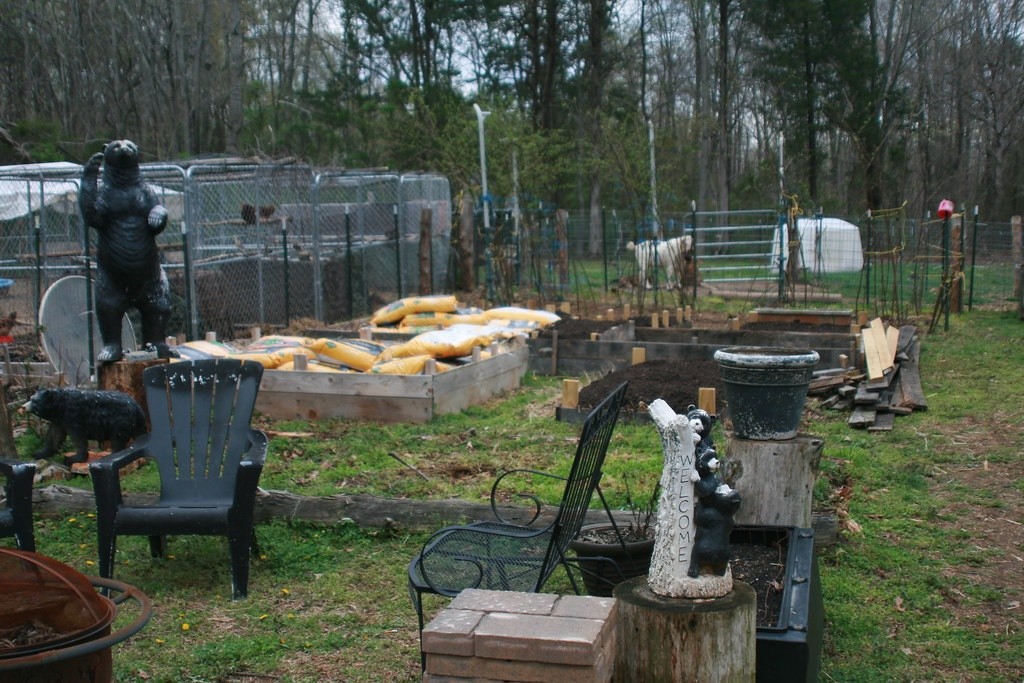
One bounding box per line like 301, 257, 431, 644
627, 235, 697, 291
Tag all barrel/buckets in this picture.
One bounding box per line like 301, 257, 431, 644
714, 346, 819, 440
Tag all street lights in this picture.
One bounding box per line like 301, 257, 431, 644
648, 119, 659, 236
473, 103, 492, 228
779, 131, 785, 205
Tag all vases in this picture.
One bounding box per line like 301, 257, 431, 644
569, 523, 655, 597
714, 345, 820, 441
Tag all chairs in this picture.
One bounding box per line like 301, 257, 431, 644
406, 380, 635, 675
89, 358, 269, 602
0, 456, 37, 573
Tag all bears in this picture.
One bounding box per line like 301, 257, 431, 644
685, 403, 741, 578
22, 387, 149, 468
78, 138, 179, 362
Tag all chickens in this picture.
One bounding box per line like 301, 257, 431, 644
241, 203, 275, 226
